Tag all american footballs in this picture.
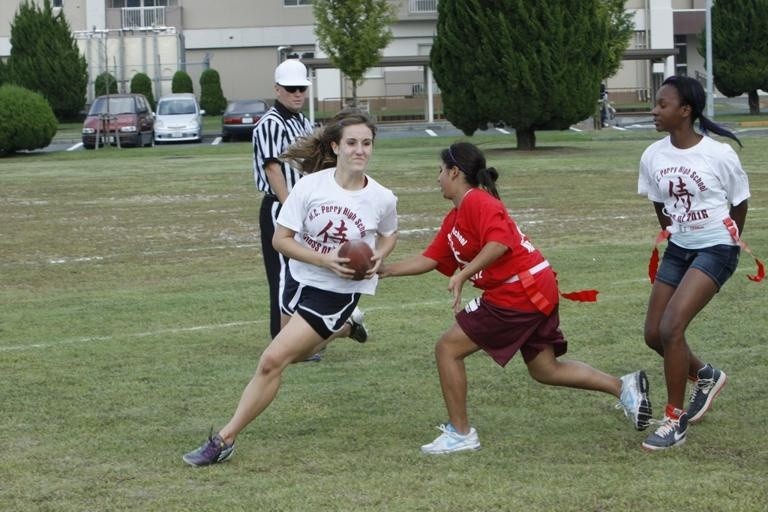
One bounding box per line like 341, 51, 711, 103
338, 240, 374, 280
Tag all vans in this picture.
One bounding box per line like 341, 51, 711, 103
81, 92, 157, 150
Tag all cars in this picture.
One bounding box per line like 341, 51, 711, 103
153, 94, 208, 144
221, 97, 271, 143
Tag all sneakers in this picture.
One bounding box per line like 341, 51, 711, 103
642, 404, 688, 451
615, 369, 653, 431
419, 424, 480, 455
183, 426, 235, 468
346, 305, 368, 343
684, 363, 727, 424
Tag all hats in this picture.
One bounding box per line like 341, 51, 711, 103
274, 59, 312, 87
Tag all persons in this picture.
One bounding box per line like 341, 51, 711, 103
639, 75, 753, 452
254, 56, 328, 364
180, 105, 398, 471
379, 141, 652, 455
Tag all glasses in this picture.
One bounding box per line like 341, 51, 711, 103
276, 82, 307, 93
